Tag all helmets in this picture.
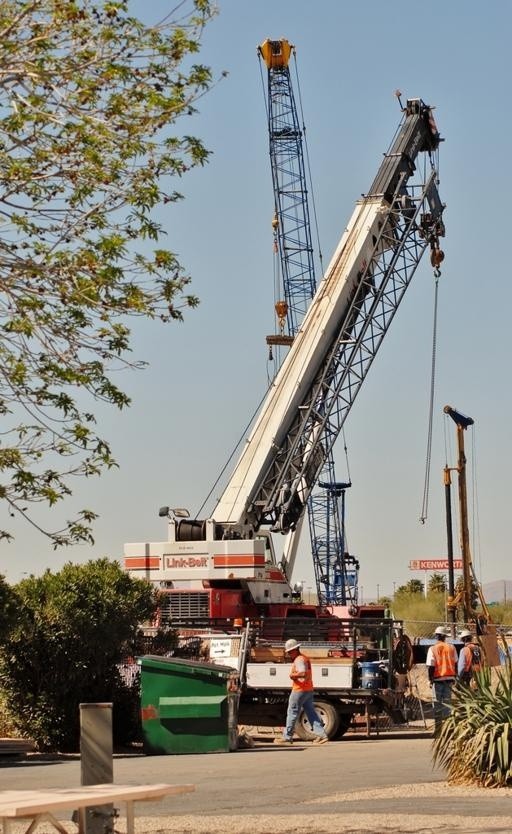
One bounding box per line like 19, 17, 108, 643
435, 625, 452, 637
459, 629, 472, 642
285, 639, 302, 653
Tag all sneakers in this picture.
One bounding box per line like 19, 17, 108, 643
274, 737, 293, 745
312, 737, 328, 744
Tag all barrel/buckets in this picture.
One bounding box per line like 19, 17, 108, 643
362, 662, 379, 688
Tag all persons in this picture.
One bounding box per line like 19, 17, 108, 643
273, 638, 329, 745
425, 625, 459, 739
455, 629, 485, 691
